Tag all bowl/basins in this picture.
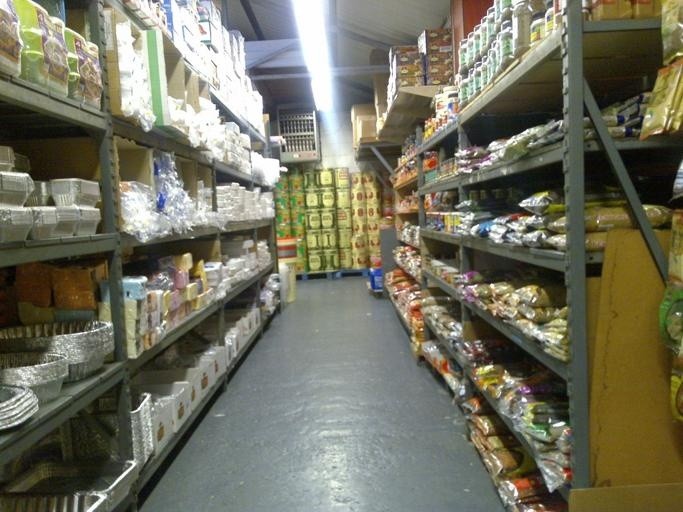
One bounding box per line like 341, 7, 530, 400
0, 351, 70, 403
0, 321, 115, 383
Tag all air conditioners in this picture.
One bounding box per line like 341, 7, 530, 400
275, 102, 322, 165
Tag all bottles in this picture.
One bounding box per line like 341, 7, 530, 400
499, 20, 515, 71
490, 40, 499, 80
458, 39, 468, 75
480, 55, 488, 92
465, 32, 477, 68
511, 1, 533, 59
468, 68, 474, 102
545, 0, 554, 38
473, 24, 482, 62
486, 48, 493, 86
472, 62, 482, 97
479, 16, 487, 58
529, 11, 544, 48
447, 92, 459, 123
459, 73, 469, 109
495, 32, 504, 75
486, 7, 496, 50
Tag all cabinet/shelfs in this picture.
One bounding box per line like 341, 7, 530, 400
0, 0, 281, 512
351, 0, 681, 512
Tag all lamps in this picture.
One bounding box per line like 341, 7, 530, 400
291, 0, 335, 116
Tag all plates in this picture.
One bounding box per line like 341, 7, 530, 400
0, 384, 38, 431
0, 492, 108, 512
4, 462, 137, 506
